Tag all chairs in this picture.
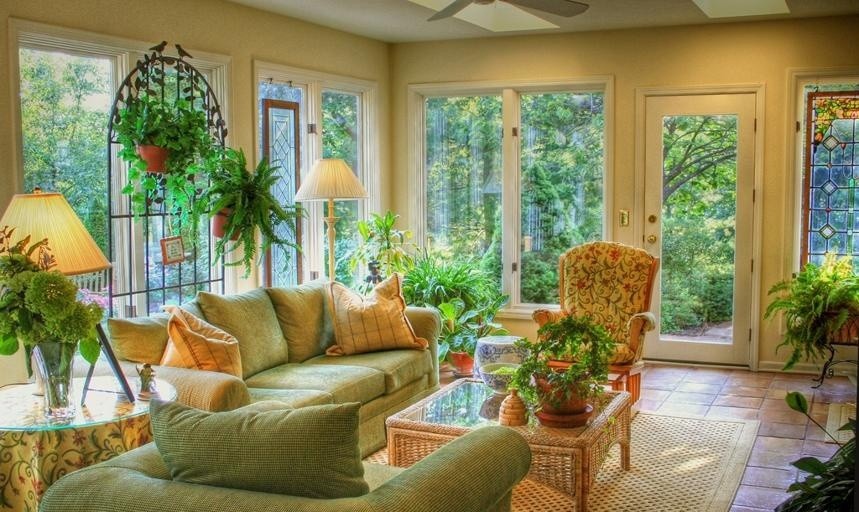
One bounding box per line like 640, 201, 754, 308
36, 395, 531, 511
530, 241, 663, 422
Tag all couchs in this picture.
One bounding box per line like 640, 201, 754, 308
105, 273, 442, 461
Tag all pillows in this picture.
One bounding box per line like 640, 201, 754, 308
146, 390, 372, 500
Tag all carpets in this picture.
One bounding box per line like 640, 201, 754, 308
359, 407, 760, 512
823, 400, 859, 444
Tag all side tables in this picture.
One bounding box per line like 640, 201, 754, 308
1, 374, 154, 511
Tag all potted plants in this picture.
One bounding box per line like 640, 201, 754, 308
434, 293, 511, 378
191, 142, 312, 280
763, 251, 859, 392
505, 310, 617, 430
108, 91, 210, 237
1, 223, 105, 407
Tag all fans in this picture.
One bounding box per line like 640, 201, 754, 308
426, 0, 591, 21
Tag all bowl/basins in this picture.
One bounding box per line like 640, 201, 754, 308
478, 362, 521, 394
479, 392, 507, 420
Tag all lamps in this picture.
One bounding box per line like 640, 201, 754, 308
1, 184, 119, 276
290, 157, 369, 282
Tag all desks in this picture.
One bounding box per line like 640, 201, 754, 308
384, 377, 633, 511
